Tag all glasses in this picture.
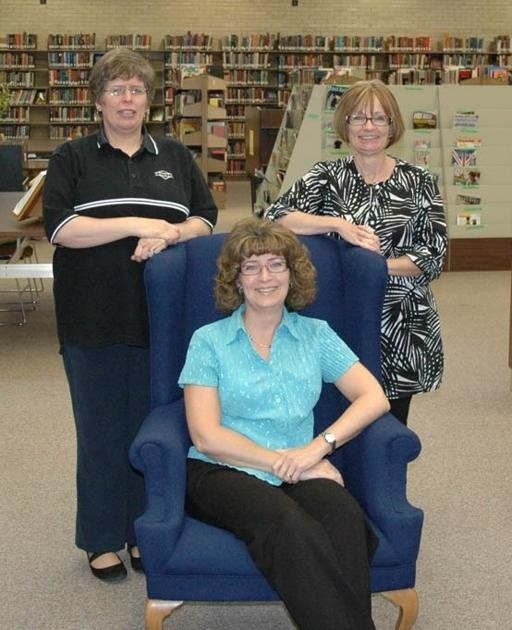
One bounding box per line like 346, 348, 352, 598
104, 86, 149, 95
239, 258, 287, 275
344, 114, 390, 125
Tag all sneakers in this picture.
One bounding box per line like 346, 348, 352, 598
87, 545, 144, 581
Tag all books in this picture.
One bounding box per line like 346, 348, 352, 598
162, 31, 436, 170
0, 32, 152, 140
431, 33, 510, 84
455, 110, 481, 226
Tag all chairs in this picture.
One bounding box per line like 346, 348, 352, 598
128, 231, 426, 630
1, 145, 47, 326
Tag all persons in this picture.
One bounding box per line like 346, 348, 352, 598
174, 214, 393, 629
261, 78, 448, 425
42, 47, 219, 580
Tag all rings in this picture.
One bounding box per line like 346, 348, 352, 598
286, 473, 292, 478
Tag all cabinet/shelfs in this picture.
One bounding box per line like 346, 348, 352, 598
2, 41, 511, 181
256, 81, 511, 274
173, 71, 227, 208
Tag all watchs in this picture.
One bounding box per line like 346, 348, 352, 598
318, 430, 337, 456
